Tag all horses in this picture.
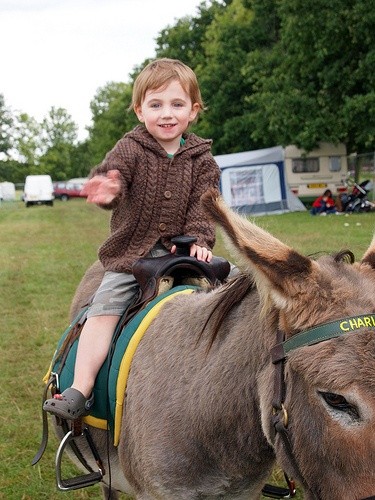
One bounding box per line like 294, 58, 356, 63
50, 189, 375, 500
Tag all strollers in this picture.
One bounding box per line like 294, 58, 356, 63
339, 177, 374, 215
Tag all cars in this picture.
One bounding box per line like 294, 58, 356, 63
54, 177, 90, 203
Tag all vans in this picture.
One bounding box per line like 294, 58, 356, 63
284, 142, 348, 202
24, 174, 54, 208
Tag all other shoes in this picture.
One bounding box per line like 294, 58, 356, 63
336, 212, 344, 215
320, 212, 326, 216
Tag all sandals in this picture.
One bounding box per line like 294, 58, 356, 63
42, 387, 94, 420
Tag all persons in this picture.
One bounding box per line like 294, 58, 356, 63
41, 58, 243, 420
318, 196, 342, 216
310, 189, 342, 216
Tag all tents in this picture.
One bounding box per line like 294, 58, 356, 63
213, 145, 307, 218
